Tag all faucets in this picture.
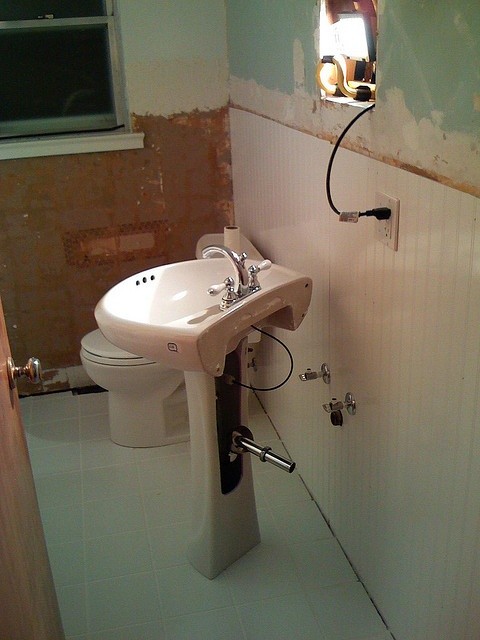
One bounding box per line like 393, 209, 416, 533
201, 244, 249, 297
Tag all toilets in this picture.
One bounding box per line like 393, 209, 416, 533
78, 233, 263, 447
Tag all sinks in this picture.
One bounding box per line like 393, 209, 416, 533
93, 255, 313, 376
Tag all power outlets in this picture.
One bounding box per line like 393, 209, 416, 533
372, 189, 401, 250
